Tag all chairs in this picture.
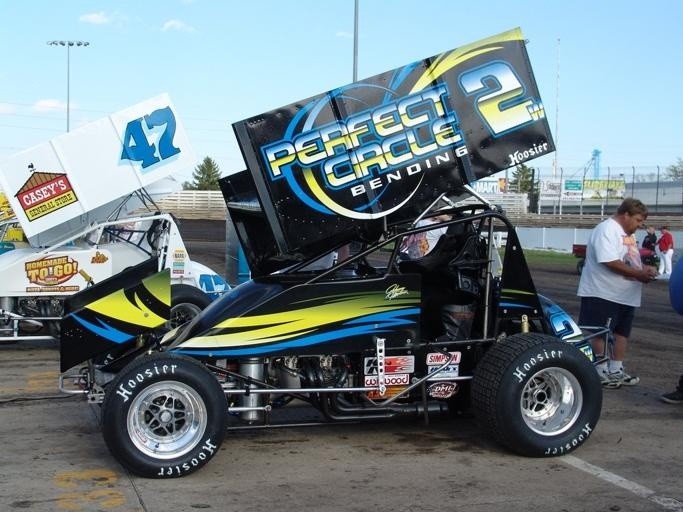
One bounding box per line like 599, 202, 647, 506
597, 371, 641, 389
660, 389, 683, 405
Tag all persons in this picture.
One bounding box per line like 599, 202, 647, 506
576, 198, 660, 390
642, 225, 657, 266
652, 228, 673, 276
660, 256, 683, 404
404, 214, 451, 260
298, 244, 352, 272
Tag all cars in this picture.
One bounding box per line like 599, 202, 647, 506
0, 204, 242, 344
61, 208, 610, 476
